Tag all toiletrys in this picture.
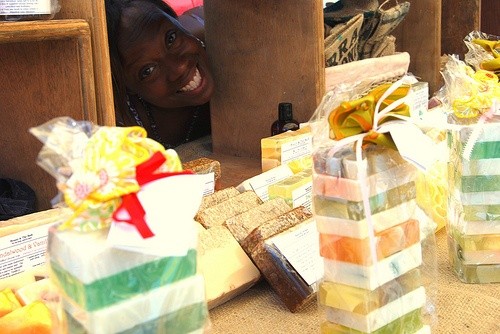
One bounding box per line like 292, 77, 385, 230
271, 103, 299, 136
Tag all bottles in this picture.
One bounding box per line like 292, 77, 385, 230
270, 102, 299, 136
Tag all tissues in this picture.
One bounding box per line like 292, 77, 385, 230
366, 76, 429, 121
